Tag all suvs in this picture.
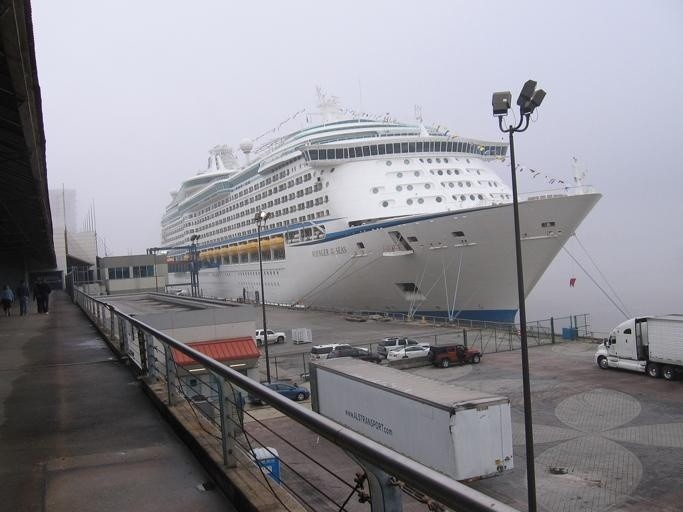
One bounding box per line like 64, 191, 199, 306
255, 329, 285, 348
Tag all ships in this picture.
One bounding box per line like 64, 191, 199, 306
153, 88, 606, 333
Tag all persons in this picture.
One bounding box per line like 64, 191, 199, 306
1, 284, 14, 318
32, 276, 52, 314
17, 280, 30, 317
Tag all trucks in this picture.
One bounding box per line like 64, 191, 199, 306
593, 315, 682, 382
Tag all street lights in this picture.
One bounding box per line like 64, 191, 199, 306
254, 208, 272, 383
490, 81, 551, 511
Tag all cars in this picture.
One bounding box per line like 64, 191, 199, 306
309, 336, 482, 368
247, 382, 309, 406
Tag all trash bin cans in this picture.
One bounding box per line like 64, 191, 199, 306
563, 327, 578, 340
249, 447, 280, 485
